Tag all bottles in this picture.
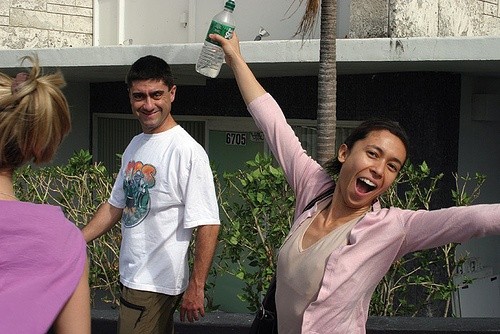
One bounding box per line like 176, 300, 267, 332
195, 1, 237, 78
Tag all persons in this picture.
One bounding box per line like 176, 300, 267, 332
0, 50, 92, 334
79, 53, 221, 334
208, 31, 500, 334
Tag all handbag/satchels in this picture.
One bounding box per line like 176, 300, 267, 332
249, 273, 278, 334
